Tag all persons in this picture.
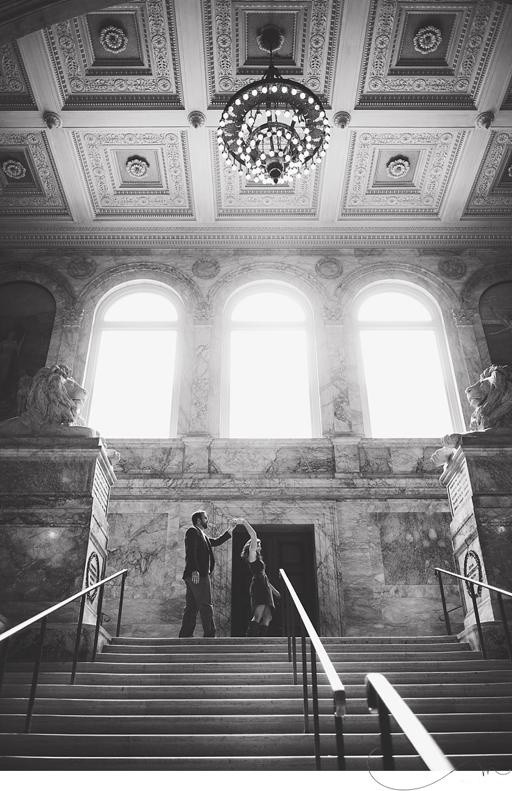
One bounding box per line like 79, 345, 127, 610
178, 511, 237, 637
231, 518, 281, 637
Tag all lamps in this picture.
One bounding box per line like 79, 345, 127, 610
208, 19, 335, 195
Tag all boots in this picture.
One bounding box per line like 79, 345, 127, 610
245, 620, 259, 637
259, 623, 269, 637
